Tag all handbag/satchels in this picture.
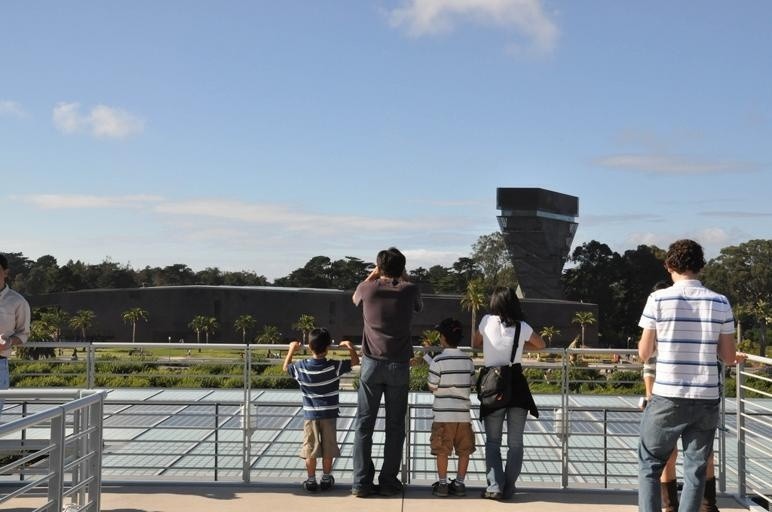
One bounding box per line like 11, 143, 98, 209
477, 362, 521, 410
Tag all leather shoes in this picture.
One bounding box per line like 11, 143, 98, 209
480, 489, 503, 499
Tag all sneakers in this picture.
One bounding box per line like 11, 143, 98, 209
303, 480, 319, 494
320, 475, 335, 489
431, 481, 448, 497
448, 478, 465, 496
351, 482, 379, 497
379, 476, 403, 497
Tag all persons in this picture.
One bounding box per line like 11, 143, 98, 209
353, 246, 423, 498
0, 254, 31, 415
426, 317, 476, 498
636, 238, 747, 511
282, 328, 359, 494
472, 287, 546, 500
641, 351, 720, 512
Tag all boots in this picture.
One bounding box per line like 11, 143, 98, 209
661, 480, 679, 511
700, 478, 719, 511
